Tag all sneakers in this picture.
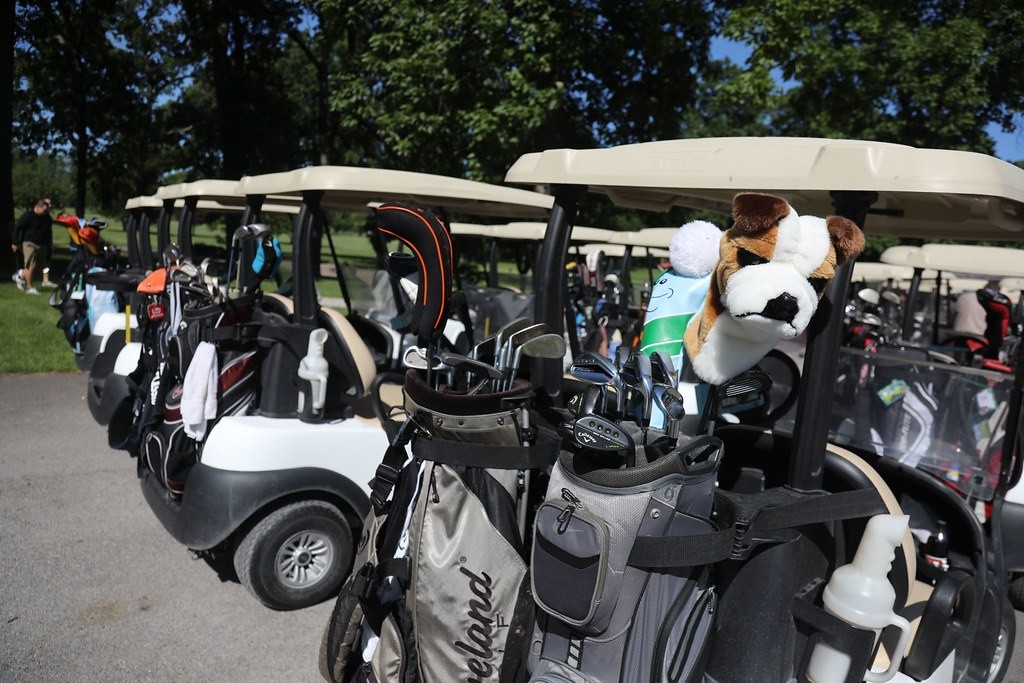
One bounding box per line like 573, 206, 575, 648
42, 280, 58, 288
12, 272, 26, 291
26, 287, 42, 296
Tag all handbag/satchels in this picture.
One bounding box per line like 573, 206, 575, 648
523, 421, 725, 683
319, 374, 533, 683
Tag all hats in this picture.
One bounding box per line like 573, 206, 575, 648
78, 226, 98, 246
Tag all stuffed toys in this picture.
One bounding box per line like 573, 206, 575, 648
640, 192, 865, 420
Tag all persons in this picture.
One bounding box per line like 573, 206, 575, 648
12, 196, 58, 296
